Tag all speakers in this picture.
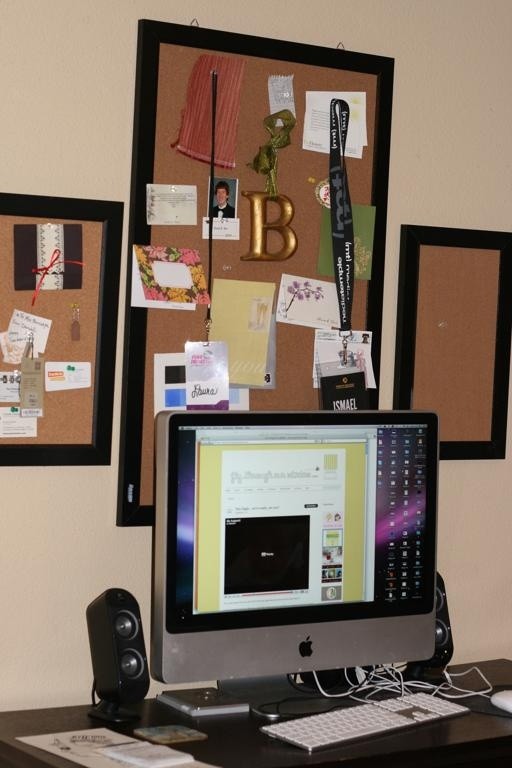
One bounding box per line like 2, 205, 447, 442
407, 568, 453, 683
86, 589, 149, 724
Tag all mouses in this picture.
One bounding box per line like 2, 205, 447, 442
489, 688, 512, 716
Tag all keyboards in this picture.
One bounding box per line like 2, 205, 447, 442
260, 692, 473, 753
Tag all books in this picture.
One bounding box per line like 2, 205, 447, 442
134, 724, 205, 743
96, 739, 194, 768
154, 687, 252, 717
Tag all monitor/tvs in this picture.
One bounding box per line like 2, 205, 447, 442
150, 411, 437, 717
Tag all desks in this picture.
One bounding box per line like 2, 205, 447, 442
0, 659, 512, 768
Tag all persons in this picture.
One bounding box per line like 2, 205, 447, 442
213, 181, 235, 218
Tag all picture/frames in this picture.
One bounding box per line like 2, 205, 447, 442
392, 223, 512, 460
0, 192, 125, 467
114, 18, 395, 528
